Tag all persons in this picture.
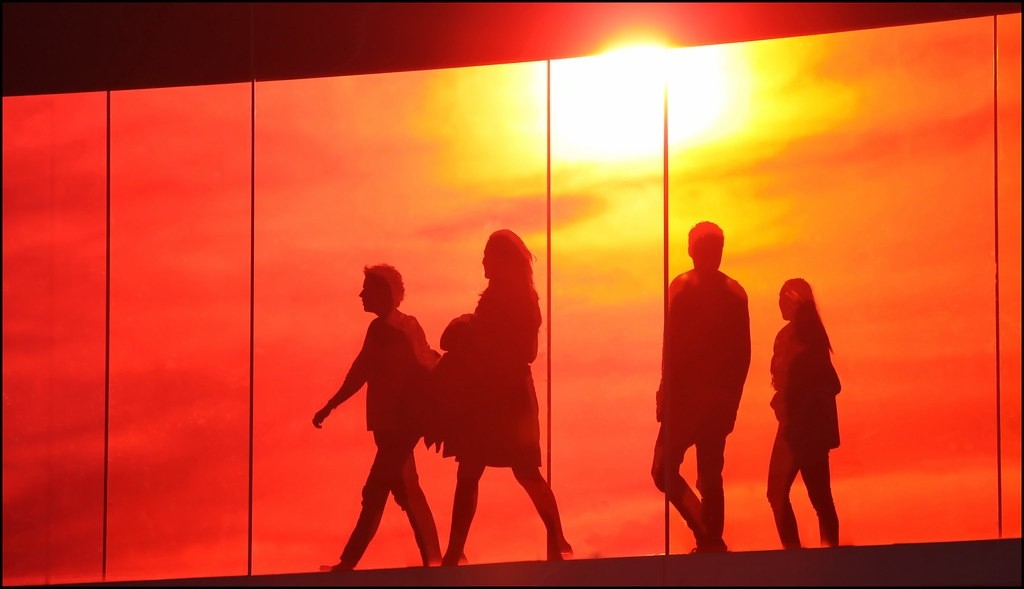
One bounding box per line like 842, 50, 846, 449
767, 278, 841, 551
438, 229, 574, 565
312, 264, 443, 571
650, 221, 750, 556
